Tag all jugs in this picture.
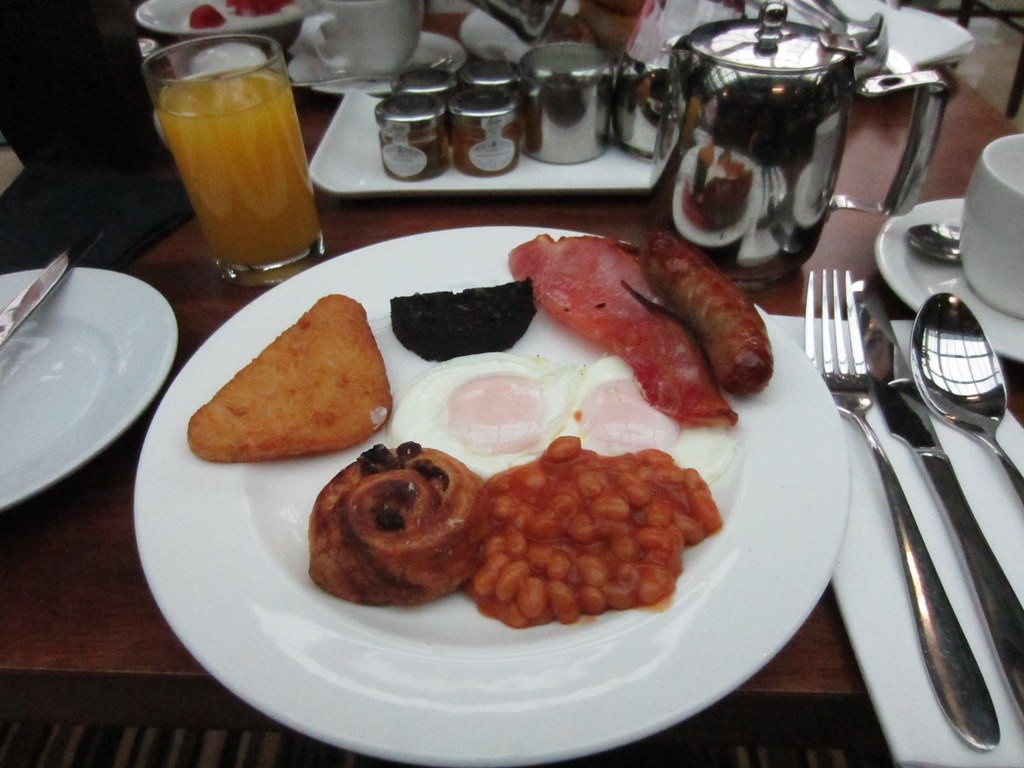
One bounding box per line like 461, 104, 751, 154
649, 0, 950, 292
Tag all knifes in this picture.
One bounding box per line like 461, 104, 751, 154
0, 226, 107, 354
849, 278, 1024, 718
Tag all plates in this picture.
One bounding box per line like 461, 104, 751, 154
133, 0, 303, 35
133, 224, 849, 768
458, 0, 580, 65
0, 267, 178, 513
288, 31, 466, 94
874, 198, 1024, 364
306, 82, 681, 201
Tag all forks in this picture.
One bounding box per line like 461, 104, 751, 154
806, 269, 1000, 749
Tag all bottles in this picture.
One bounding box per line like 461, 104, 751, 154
375, 43, 613, 181
614, 0, 742, 162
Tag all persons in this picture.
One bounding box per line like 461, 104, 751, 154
422, 0, 643, 62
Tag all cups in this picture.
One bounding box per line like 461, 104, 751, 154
141, 36, 325, 287
301, 0, 423, 76
960, 133, 1024, 321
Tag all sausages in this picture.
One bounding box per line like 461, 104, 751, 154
642, 229, 775, 394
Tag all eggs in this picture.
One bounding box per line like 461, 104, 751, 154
383, 351, 577, 479
574, 356, 734, 483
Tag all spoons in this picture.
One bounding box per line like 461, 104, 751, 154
904, 224, 961, 262
910, 293, 1024, 509
